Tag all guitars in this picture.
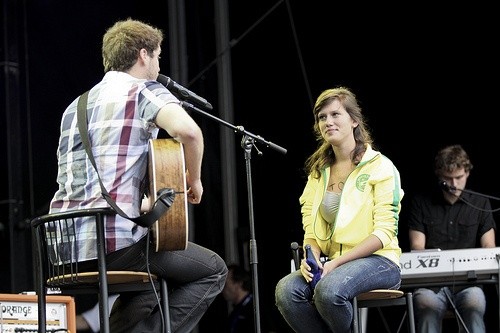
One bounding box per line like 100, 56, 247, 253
140, 137, 188, 252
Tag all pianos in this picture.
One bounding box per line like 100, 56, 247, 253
291, 247, 500, 333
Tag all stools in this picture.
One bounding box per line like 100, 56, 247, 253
353, 288, 415, 333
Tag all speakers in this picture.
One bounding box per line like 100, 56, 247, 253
0, 293, 76, 333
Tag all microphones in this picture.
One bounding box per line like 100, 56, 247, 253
440, 182, 453, 194
157, 74, 214, 111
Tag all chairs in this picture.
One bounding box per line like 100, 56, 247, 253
30, 208, 173, 333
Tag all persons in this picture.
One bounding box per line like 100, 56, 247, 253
275, 88, 404, 333
76, 294, 120, 333
407, 146, 496, 333
45, 20, 227, 333
223, 264, 255, 333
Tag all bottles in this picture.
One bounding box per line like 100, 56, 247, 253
305, 244, 322, 290
